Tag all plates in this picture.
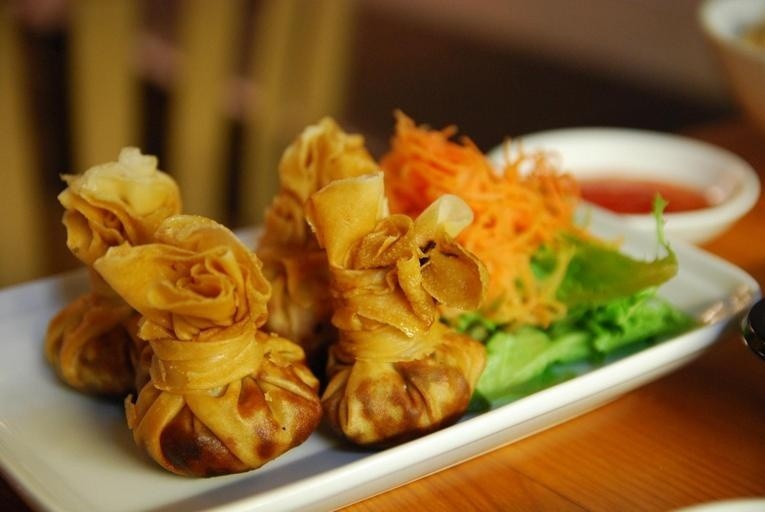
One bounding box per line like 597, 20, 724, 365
0, 197, 764, 510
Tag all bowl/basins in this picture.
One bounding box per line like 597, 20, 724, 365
482, 126, 760, 249
